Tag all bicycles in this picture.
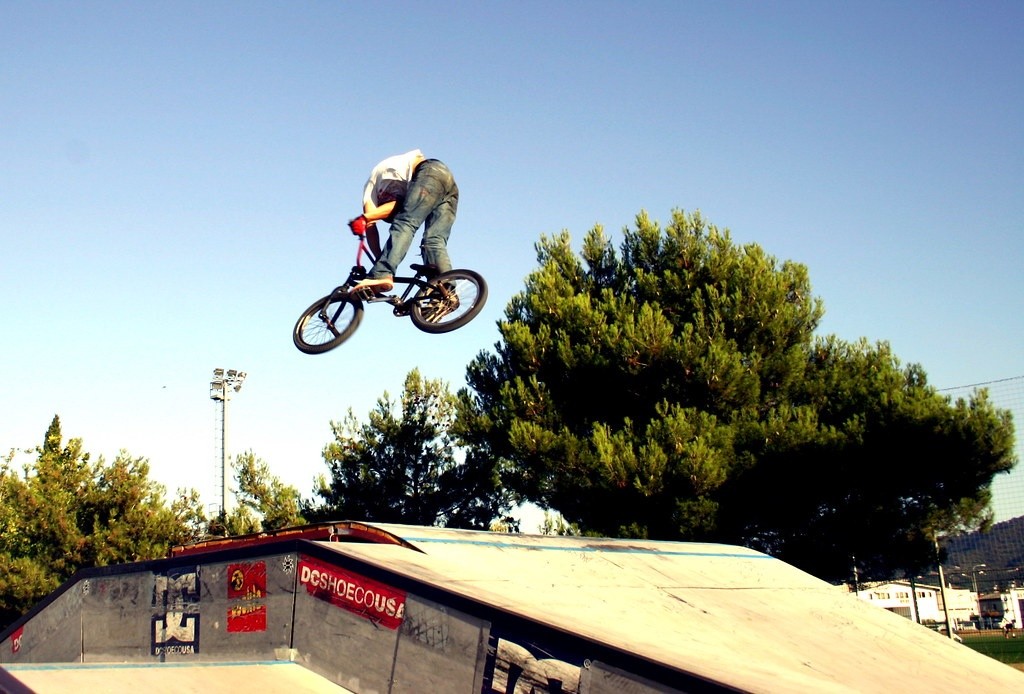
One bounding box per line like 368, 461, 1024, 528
293, 212, 490, 357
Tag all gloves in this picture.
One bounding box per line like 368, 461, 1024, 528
348, 214, 368, 235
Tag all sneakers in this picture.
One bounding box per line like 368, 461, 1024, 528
349, 277, 393, 301
420, 295, 459, 319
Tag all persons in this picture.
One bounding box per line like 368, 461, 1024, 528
970, 608, 1017, 640
347, 150, 458, 323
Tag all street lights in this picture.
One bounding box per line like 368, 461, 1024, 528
934, 531, 955, 642
209, 367, 247, 536
973, 564, 987, 620
